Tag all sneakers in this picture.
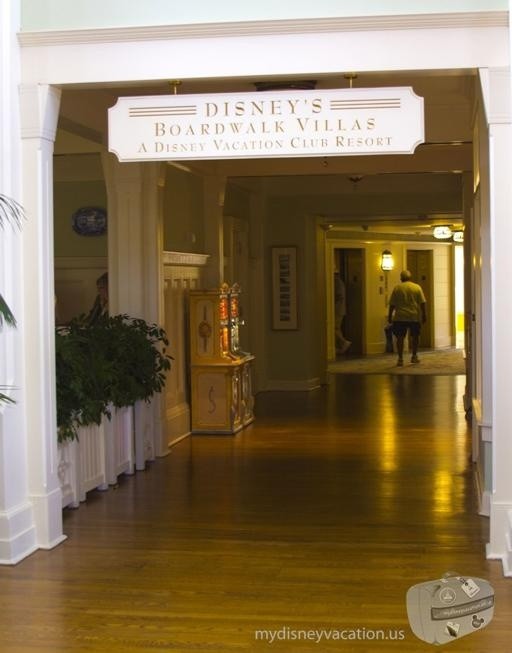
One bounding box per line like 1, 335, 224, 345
341, 341, 352, 352
410, 356, 419, 362
396, 359, 404, 366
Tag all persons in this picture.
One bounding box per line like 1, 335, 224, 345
95, 271, 107, 311
334, 270, 354, 358
388, 269, 426, 366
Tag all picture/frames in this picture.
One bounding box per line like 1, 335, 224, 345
270, 245, 300, 332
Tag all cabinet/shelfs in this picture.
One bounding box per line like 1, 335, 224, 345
184, 282, 258, 437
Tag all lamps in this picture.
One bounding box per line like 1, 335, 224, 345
431, 224, 464, 243
381, 249, 393, 272
